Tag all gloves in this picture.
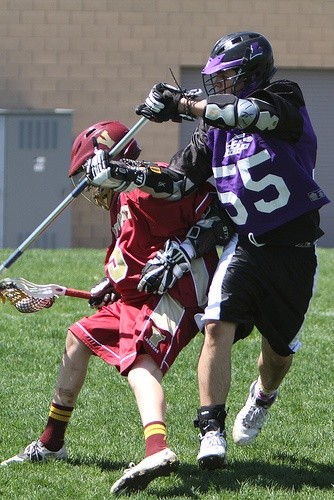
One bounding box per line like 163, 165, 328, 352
135, 83, 187, 123
137, 239, 191, 295
85, 149, 137, 193
88, 276, 122, 309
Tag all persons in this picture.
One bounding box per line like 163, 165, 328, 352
1, 120, 233, 498
85, 30, 332, 468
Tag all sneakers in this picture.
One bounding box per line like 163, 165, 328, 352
231, 379, 279, 447
110, 448, 180, 497
1, 440, 67, 466
196, 428, 228, 470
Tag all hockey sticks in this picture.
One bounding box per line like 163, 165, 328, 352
0, 83, 181, 276
0, 278, 93, 314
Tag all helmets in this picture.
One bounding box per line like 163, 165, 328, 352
68, 120, 141, 178
201, 31, 278, 99
